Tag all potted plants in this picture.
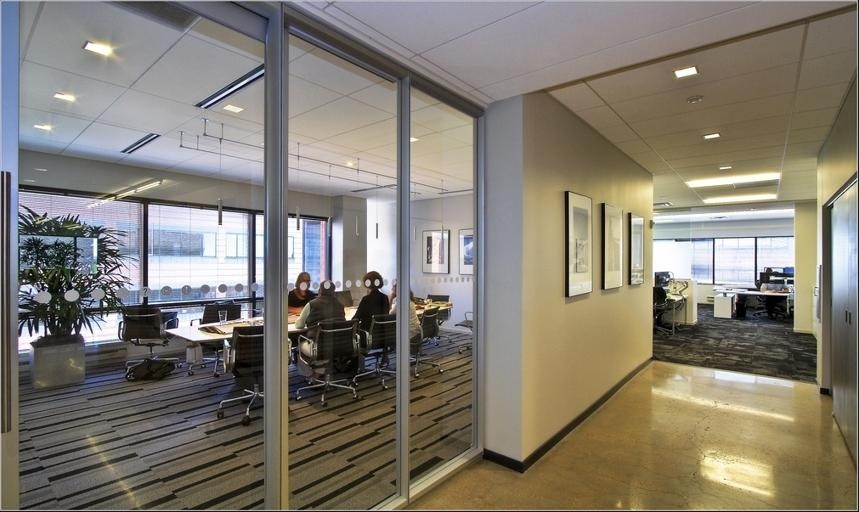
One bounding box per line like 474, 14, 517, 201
18, 202, 141, 391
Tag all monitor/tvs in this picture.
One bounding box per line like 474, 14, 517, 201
655, 272, 670, 287
783, 267, 794, 273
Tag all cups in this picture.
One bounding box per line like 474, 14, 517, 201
218, 310, 228, 327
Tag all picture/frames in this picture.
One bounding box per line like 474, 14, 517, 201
423, 229, 474, 275
564, 192, 645, 299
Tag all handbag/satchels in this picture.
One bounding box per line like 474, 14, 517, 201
123, 358, 175, 382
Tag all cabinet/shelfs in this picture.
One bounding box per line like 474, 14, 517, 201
714, 297, 733, 320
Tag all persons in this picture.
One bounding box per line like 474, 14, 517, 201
378, 279, 422, 368
334, 270, 390, 374
286, 271, 319, 314
294, 279, 346, 385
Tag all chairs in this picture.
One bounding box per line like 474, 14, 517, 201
653, 287, 673, 338
753, 272, 793, 320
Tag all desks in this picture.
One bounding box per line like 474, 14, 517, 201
653, 293, 688, 336
712, 290, 794, 315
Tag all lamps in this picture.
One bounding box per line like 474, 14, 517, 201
114, 176, 163, 213
179, 117, 452, 244
